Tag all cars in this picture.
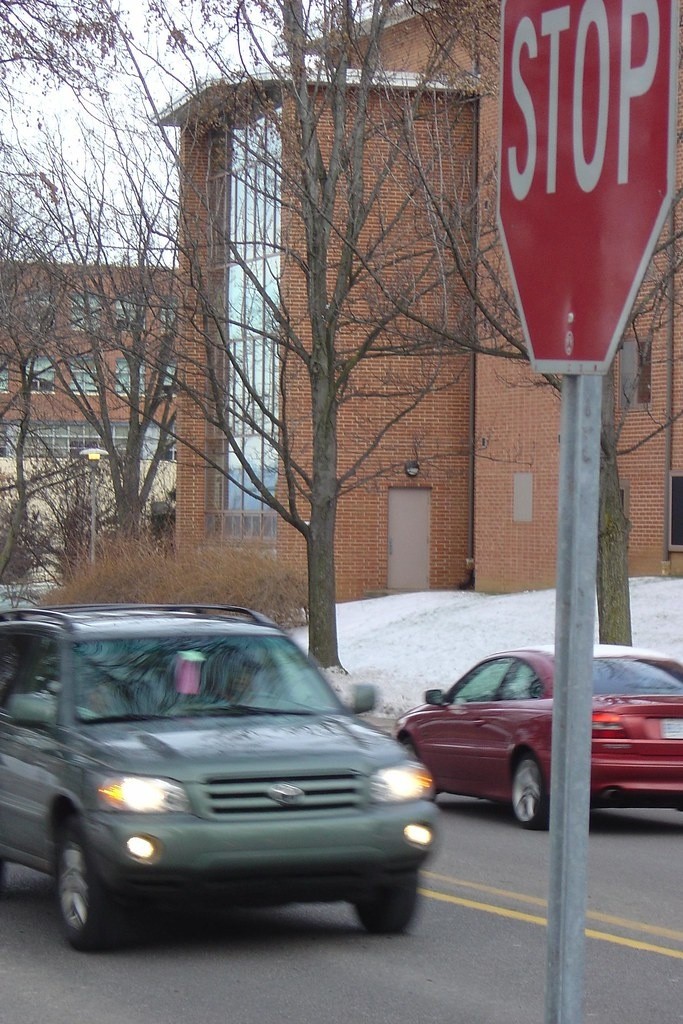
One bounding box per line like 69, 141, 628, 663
393, 643, 683, 828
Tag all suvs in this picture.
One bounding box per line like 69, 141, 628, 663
1, 602, 442, 952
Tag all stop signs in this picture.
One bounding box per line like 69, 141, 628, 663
494, 1, 683, 378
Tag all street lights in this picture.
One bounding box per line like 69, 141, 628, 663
79, 442, 110, 574
